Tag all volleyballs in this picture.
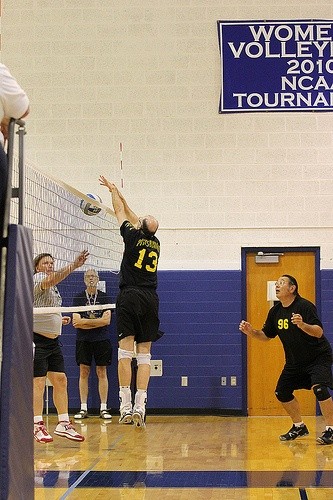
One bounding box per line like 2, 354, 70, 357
80, 192, 102, 216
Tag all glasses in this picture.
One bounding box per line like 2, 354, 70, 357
84, 275, 98, 279
274, 282, 291, 287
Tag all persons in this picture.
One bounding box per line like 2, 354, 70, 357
0, 64, 31, 253
239, 275, 333, 444
32, 250, 90, 443
99, 175, 165, 433
72, 269, 114, 420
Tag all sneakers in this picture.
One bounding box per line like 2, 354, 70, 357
100, 409, 113, 419
315, 427, 333, 444
74, 409, 88, 419
118, 411, 133, 424
132, 410, 146, 428
53, 421, 85, 442
33, 420, 53, 443
279, 423, 310, 441
